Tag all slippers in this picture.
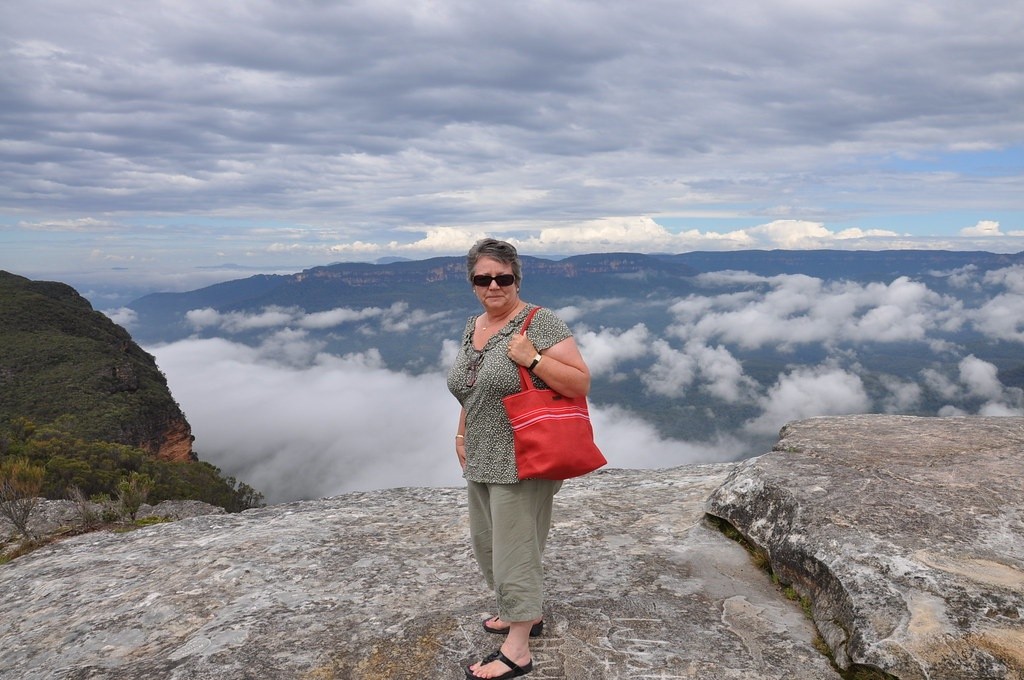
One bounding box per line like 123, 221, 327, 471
481, 614, 544, 636
464, 651, 532, 680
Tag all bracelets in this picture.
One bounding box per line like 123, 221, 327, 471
455, 435, 464, 438
528, 353, 541, 370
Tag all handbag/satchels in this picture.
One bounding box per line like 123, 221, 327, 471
501, 308, 606, 481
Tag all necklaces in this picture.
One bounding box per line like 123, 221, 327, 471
483, 317, 496, 330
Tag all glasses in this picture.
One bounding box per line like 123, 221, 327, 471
470, 274, 515, 287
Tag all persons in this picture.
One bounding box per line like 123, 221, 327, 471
447, 238, 591, 680
